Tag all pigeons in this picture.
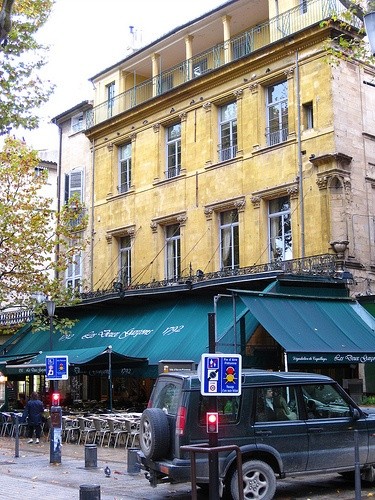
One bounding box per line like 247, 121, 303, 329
104, 466, 111, 477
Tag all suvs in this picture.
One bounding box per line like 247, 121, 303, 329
137, 369, 375, 500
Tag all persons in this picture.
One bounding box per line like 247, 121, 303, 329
12, 393, 28, 410
105, 390, 146, 411
61, 392, 73, 406
21, 392, 44, 444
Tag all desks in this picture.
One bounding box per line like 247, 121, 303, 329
72, 411, 88, 415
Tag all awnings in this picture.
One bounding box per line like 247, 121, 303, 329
0, 281, 280, 378
233, 289, 375, 363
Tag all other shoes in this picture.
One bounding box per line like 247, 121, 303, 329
27, 439, 33, 444
35, 438, 39, 444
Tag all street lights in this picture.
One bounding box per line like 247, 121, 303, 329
46, 297, 57, 393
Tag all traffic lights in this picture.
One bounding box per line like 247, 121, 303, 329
52, 393, 60, 406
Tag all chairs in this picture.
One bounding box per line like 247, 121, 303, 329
272, 394, 296, 419
257, 393, 266, 420
1, 409, 142, 450
